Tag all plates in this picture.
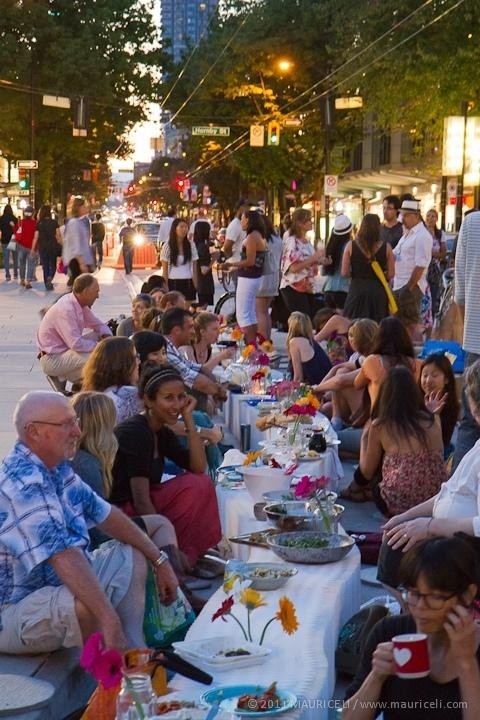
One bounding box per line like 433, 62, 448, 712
172, 635, 270, 664
200, 686, 295, 717
326, 438, 343, 447
298, 454, 326, 461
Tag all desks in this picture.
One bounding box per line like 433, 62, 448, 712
121, 321, 366, 720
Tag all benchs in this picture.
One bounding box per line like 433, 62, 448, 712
2, 639, 95, 720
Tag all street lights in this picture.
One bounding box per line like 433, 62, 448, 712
441, 98, 480, 235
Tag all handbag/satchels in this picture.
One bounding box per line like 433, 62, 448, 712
16, 231, 22, 241
81, 646, 170, 720
142, 560, 196, 650
384, 282, 398, 315
262, 249, 279, 275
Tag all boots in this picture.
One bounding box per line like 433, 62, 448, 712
159, 545, 205, 614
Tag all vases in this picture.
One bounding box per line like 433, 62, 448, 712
310, 500, 339, 535
114, 667, 154, 719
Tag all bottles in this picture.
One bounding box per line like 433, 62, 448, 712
115, 671, 158, 720
240, 424, 251, 450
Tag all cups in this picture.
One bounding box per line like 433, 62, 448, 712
390, 635, 432, 683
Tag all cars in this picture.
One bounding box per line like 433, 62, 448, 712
131, 221, 163, 253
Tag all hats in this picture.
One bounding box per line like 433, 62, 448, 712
333, 215, 352, 235
398, 201, 417, 213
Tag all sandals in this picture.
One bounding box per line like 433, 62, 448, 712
340, 482, 373, 503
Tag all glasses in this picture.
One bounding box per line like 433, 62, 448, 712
401, 588, 454, 611
24, 415, 82, 429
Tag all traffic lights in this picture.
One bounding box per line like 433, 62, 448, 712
18, 168, 31, 191
175, 177, 186, 190
267, 122, 280, 146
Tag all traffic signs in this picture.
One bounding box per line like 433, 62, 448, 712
192, 126, 230, 137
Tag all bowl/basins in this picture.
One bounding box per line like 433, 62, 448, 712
239, 562, 298, 590
236, 465, 292, 499
263, 503, 345, 530
264, 490, 339, 503
267, 530, 356, 565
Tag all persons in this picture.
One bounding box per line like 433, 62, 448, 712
1, 194, 480, 720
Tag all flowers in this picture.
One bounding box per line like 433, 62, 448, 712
76, 628, 147, 718
283, 463, 334, 537
216, 318, 321, 468
199, 566, 300, 647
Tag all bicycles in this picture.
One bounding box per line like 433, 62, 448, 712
213, 290, 237, 326
213, 245, 237, 292
